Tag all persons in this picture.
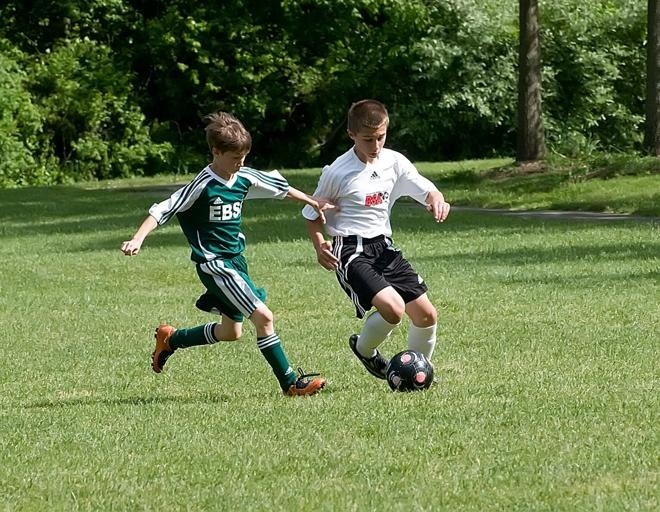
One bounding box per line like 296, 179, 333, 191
299, 98, 452, 384
119, 108, 340, 399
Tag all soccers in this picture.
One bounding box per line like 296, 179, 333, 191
386, 350, 439, 394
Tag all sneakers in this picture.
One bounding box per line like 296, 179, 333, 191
283, 376, 324, 396
350, 333, 388, 379
150, 324, 176, 373
